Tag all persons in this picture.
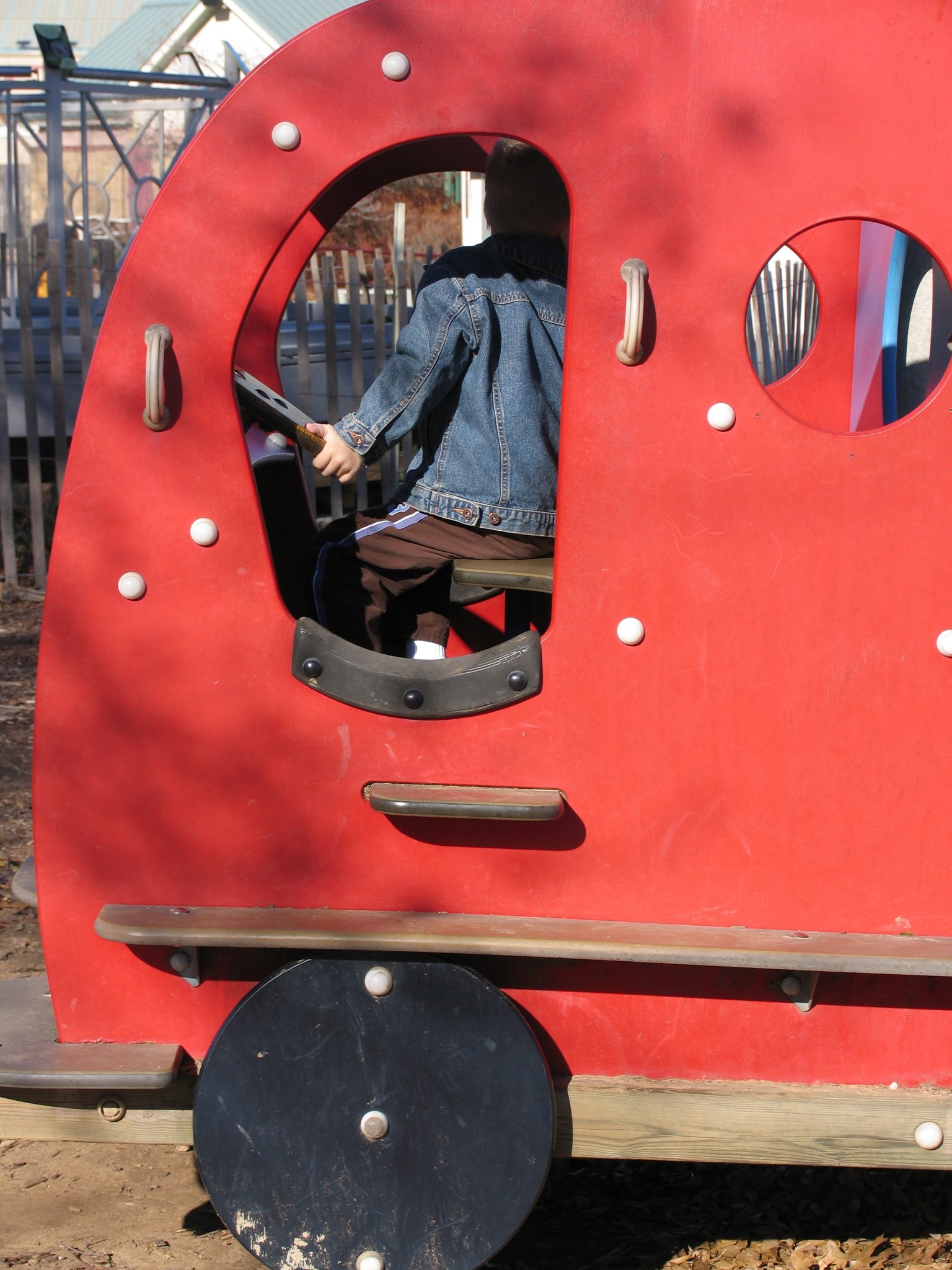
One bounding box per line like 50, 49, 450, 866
306, 136, 570, 660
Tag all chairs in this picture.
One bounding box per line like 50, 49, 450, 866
451, 556, 553, 643
